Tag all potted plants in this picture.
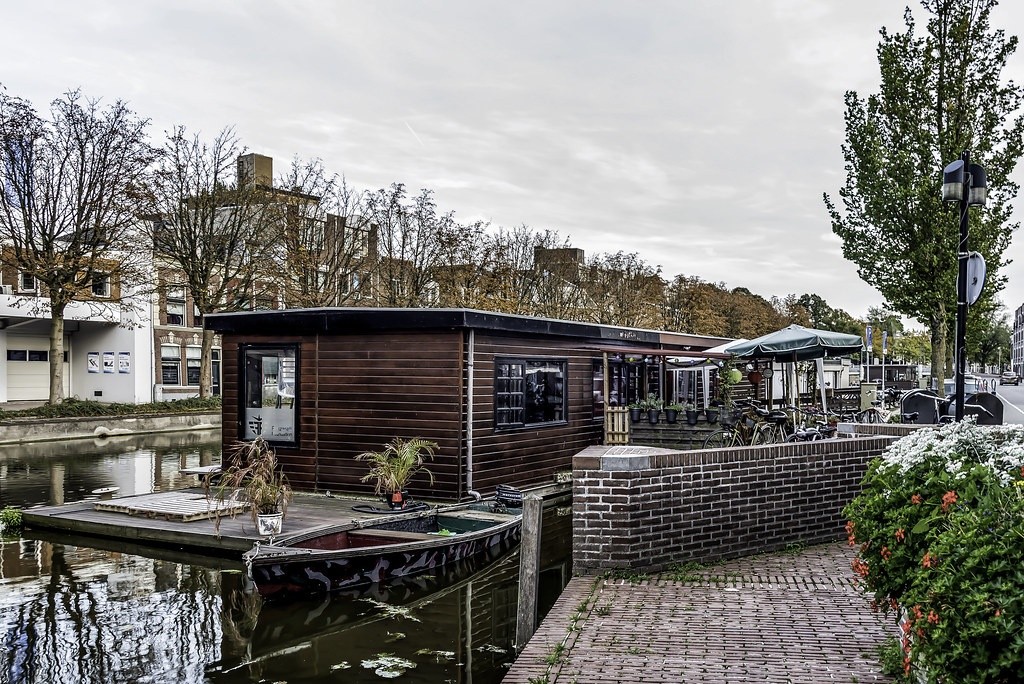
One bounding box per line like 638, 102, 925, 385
642, 392, 664, 423
627, 403, 643, 422
553, 470, 572, 484
665, 403, 683, 422
199, 435, 293, 540
703, 406, 719, 423
353, 435, 441, 511
687, 405, 702, 425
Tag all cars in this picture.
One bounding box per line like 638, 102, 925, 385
999, 371, 1019, 386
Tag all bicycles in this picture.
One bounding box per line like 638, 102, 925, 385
700, 395, 883, 451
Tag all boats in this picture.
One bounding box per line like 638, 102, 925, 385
240, 482, 525, 606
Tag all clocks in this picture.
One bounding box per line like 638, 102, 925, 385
763, 368, 774, 378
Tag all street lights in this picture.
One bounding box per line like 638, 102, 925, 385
942, 149, 988, 421
